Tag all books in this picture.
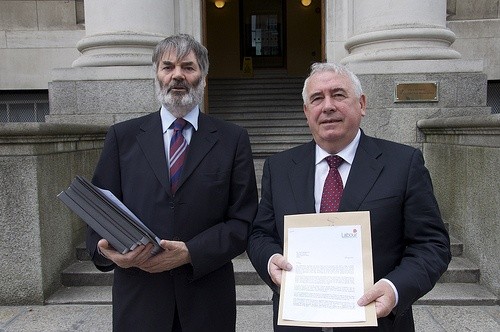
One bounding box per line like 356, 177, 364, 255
57, 175, 165, 256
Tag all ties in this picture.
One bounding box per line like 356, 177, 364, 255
319, 155, 345, 213
169, 118, 189, 191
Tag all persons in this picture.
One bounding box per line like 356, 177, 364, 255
86, 34, 259, 332
245, 61, 452, 332
